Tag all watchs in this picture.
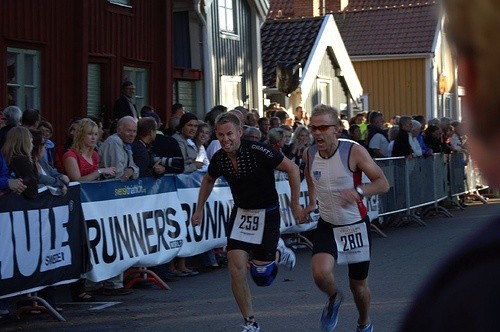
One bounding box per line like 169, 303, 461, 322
356, 185, 365, 200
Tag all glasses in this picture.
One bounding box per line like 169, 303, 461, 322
307, 124, 336, 132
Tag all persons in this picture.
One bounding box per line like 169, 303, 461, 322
191, 111, 314, 332
402, 0, 500, 332
301, 104, 390, 332
0, 105, 70, 324
61, 81, 470, 303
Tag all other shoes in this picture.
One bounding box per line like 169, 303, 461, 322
355, 320, 373, 332
285, 238, 294, 245
189, 270, 200, 275
318, 291, 344, 332
241, 323, 260, 332
84, 288, 104, 294
73, 294, 96, 302
178, 272, 189, 277
211, 263, 222, 269
103, 286, 128, 294
277, 238, 297, 270
